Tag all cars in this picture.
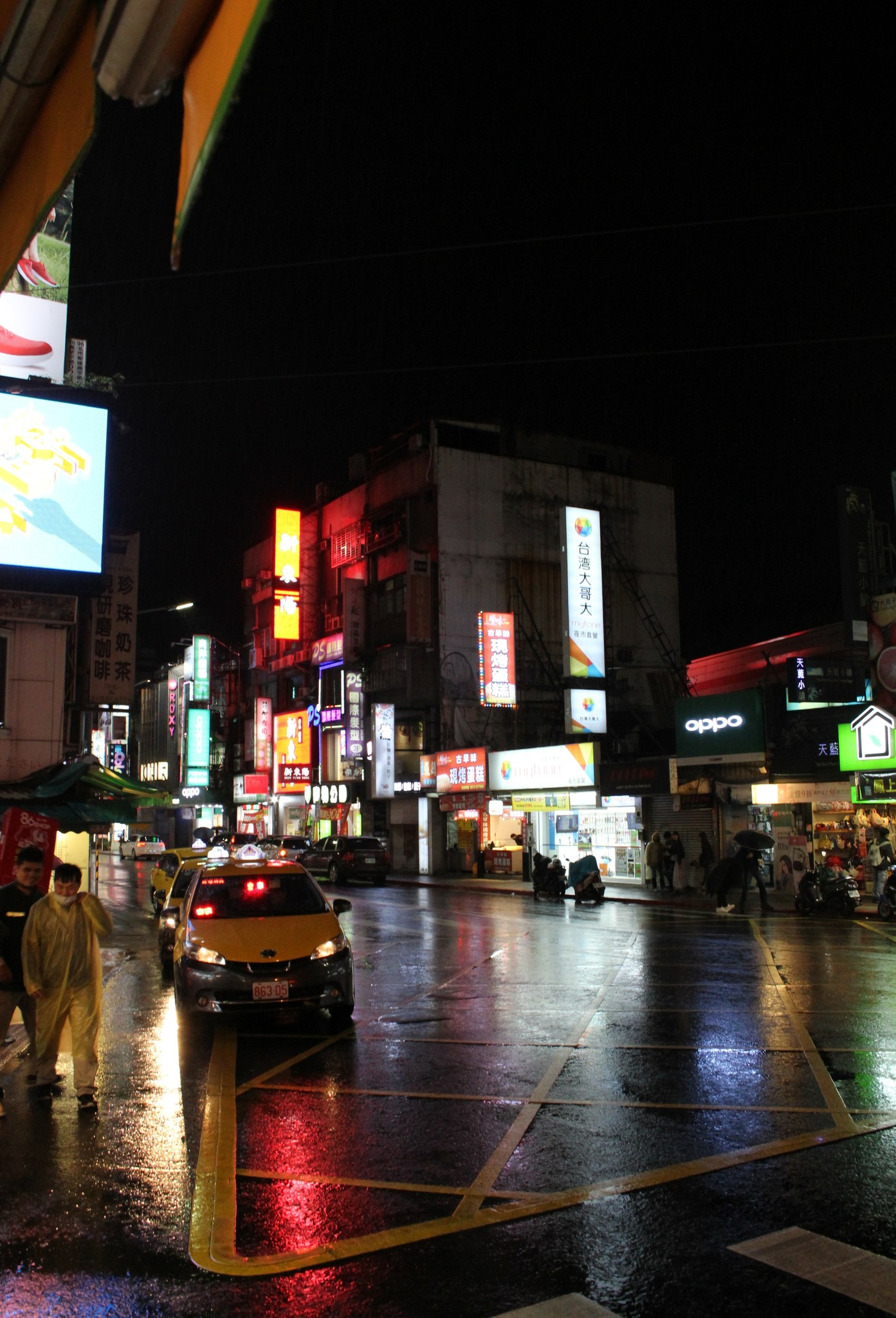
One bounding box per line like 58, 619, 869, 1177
149, 838, 355, 1024
119, 834, 165, 861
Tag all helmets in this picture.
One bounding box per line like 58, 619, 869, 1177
553, 859, 562, 868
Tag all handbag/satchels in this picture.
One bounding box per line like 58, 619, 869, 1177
816, 802, 883, 870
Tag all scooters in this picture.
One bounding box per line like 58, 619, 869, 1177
532, 849, 566, 901
794, 866, 823, 914
565, 858, 606, 904
877, 857, 896, 921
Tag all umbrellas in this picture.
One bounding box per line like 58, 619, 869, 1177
193, 827, 214, 839
733, 830, 775, 850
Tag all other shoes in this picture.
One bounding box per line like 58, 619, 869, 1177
75, 1093, 97, 1106
37, 1084, 53, 1100
25, 1041, 35, 1053
0, 1035, 16, 1046
873, 897, 880, 902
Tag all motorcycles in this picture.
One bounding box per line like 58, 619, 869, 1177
817, 851, 862, 912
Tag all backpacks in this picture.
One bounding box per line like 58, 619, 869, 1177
868, 838, 891, 866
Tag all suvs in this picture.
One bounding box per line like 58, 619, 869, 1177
209, 832, 389, 888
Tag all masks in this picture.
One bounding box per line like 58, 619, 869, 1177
55, 894, 76, 906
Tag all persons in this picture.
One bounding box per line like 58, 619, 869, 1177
661, 831, 694, 894
0, 846, 62, 1083
697, 831, 714, 893
16, 207, 58, 285
644, 832, 668, 891
511, 834, 522, 845
868, 827, 896, 903
738, 847, 774, 910
705, 850, 752, 913
776, 855, 796, 894
22, 863, 112, 1109
